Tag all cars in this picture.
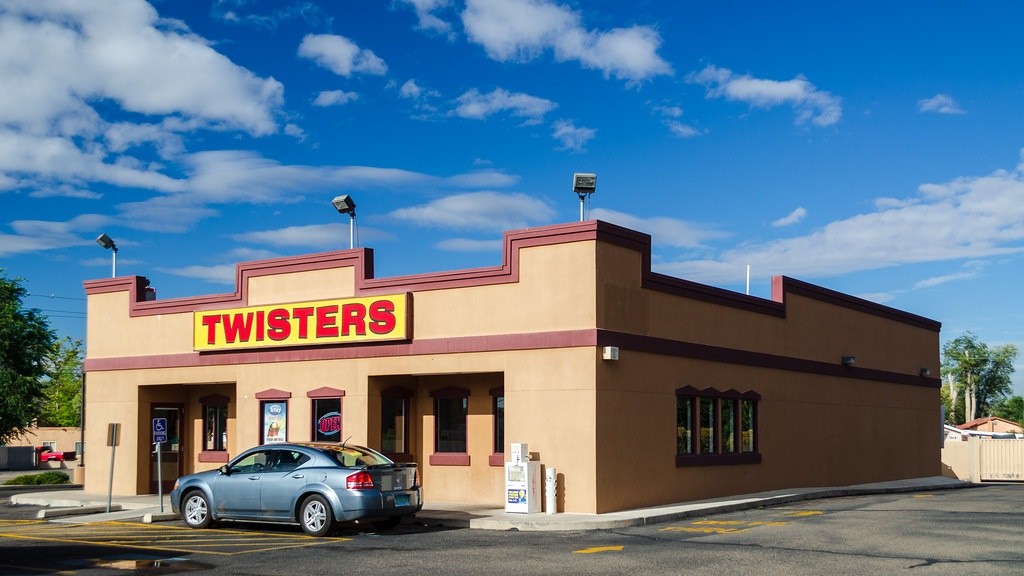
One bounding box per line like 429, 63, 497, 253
36, 446, 63, 463
171, 441, 424, 539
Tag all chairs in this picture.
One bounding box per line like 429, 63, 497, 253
277, 451, 296, 469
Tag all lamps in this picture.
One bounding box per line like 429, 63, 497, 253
841, 354, 855, 365
918, 367, 931, 375
